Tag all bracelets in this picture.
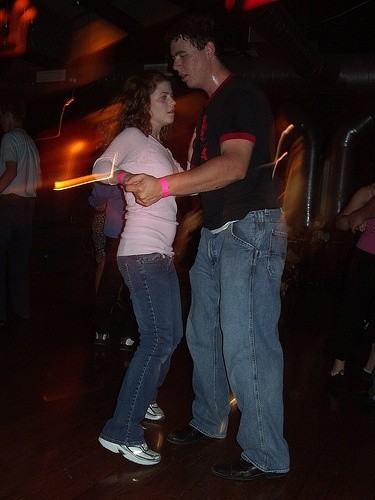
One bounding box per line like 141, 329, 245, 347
118, 171, 125, 184
160, 178, 169, 198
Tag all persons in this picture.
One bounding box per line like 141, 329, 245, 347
173, 106, 305, 412
88, 181, 139, 359
0, 96, 41, 325
329, 184, 375, 383
91, 68, 198, 465
121, 18, 292, 480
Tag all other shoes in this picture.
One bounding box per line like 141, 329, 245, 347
329, 368, 344, 395
365, 372, 375, 406
359, 368, 373, 381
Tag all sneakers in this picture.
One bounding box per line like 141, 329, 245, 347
142, 403, 166, 422
99, 431, 161, 465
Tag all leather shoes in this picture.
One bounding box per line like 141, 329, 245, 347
166, 424, 207, 444
213, 461, 290, 481
